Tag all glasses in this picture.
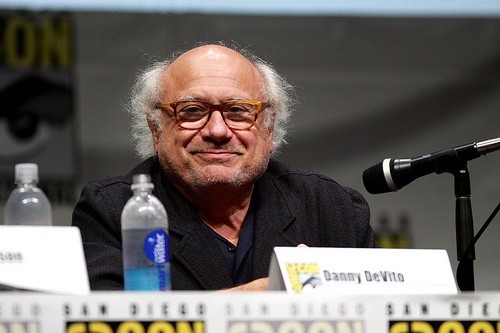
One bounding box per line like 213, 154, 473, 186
151, 98, 270, 131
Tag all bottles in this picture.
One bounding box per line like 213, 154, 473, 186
121, 175, 172, 290
4, 163, 53, 226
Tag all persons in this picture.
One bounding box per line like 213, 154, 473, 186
70, 42, 381, 291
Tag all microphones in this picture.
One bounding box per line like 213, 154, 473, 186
363, 137, 500, 195
